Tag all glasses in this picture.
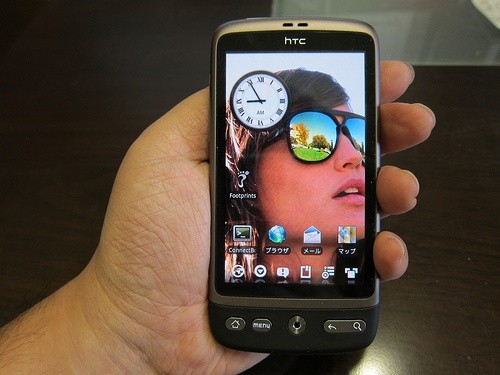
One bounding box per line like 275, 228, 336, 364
261, 107, 367, 164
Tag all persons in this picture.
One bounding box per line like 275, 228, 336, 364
0, 58, 438, 375
225, 68, 366, 283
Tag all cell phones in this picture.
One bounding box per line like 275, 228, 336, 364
205, 15, 383, 359
